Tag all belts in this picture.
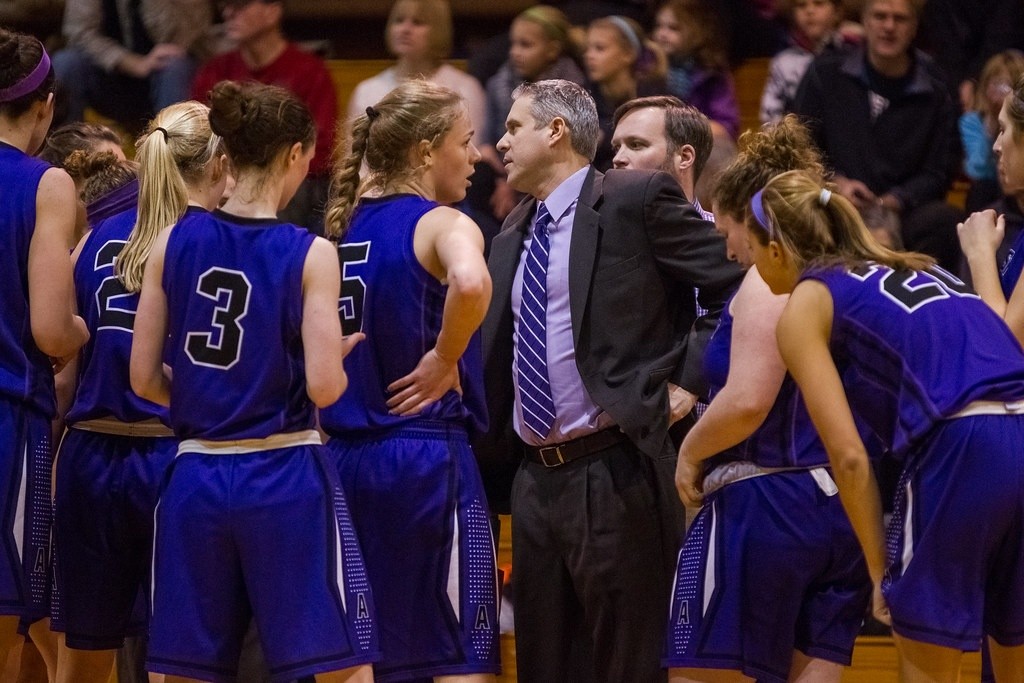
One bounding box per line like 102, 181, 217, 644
524, 424, 626, 470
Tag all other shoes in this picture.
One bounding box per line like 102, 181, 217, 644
499, 585, 514, 634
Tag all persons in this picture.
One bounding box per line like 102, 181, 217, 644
0, 34, 492, 683
0, 1, 1023, 276
660, 81, 1024, 683
471, 80, 750, 683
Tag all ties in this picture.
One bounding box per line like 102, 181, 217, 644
516, 201, 559, 440
